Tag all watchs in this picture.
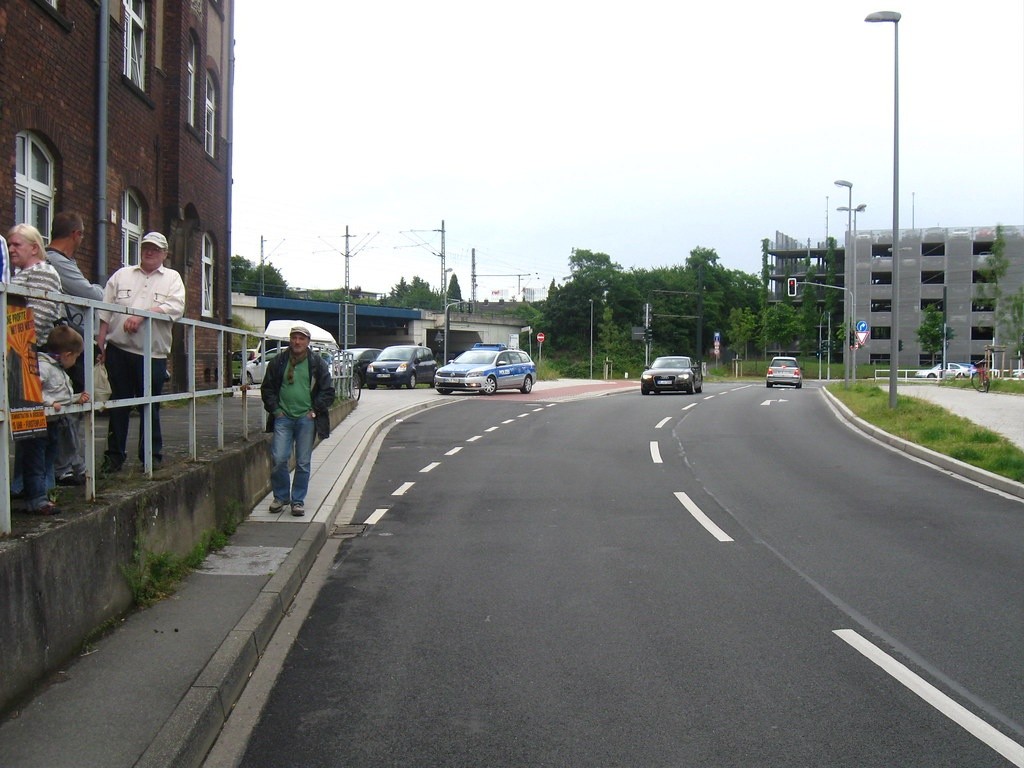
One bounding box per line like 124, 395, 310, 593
309, 411, 316, 418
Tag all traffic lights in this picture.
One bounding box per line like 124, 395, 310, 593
789, 279, 795, 294
648, 329, 653, 343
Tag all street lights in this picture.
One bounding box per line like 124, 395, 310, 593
833, 180, 867, 388
864, 10, 903, 413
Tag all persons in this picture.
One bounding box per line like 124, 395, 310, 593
97, 232, 185, 473
260, 327, 335, 515
0, 211, 104, 515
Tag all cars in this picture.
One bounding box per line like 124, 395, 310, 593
231, 349, 261, 384
330, 348, 384, 389
765, 356, 802, 389
639, 355, 704, 394
434, 343, 537, 395
915, 362, 971, 377
366, 345, 439, 390
246, 346, 334, 384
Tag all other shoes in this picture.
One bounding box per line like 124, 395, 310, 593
10, 491, 26, 500
26, 501, 62, 514
269, 497, 291, 513
55, 470, 79, 485
101, 459, 124, 472
153, 456, 161, 469
292, 503, 305, 516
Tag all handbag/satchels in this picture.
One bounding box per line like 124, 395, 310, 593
93, 360, 112, 414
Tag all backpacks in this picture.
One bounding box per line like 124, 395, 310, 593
52, 305, 103, 388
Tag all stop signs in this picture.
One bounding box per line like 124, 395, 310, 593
537, 332, 544, 342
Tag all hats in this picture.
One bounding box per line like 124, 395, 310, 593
290, 327, 311, 337
141, 232, 169, 249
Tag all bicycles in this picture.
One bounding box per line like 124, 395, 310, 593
971, 358, 991, 392
344, 361, 364, 401
